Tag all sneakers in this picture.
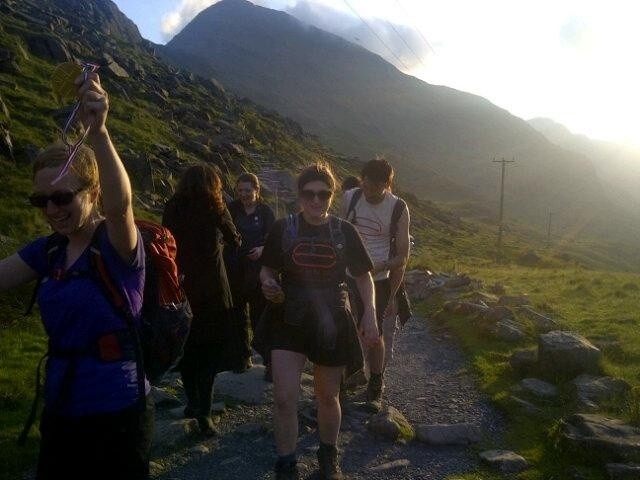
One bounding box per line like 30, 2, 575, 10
275, 455, 300, 480
337, 383, 354, 417
317, 444, 344, 480
264, 364, 273, 381
366, 377, 384, 412
184, 407, 219, 438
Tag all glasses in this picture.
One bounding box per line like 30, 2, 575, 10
30, 191, 82, 208
300, 190, 333, 202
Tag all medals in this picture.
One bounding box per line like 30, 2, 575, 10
49, 62, 85, 99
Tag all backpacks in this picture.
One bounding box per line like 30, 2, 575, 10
38, 219, 194, 388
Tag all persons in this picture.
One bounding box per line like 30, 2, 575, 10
337, 159, 413, 400
256, 159, 380, 478
160, 164, 244, 440
222, 173, 275, 384
0, 71, 156, 480
341, 174, 362, 192
